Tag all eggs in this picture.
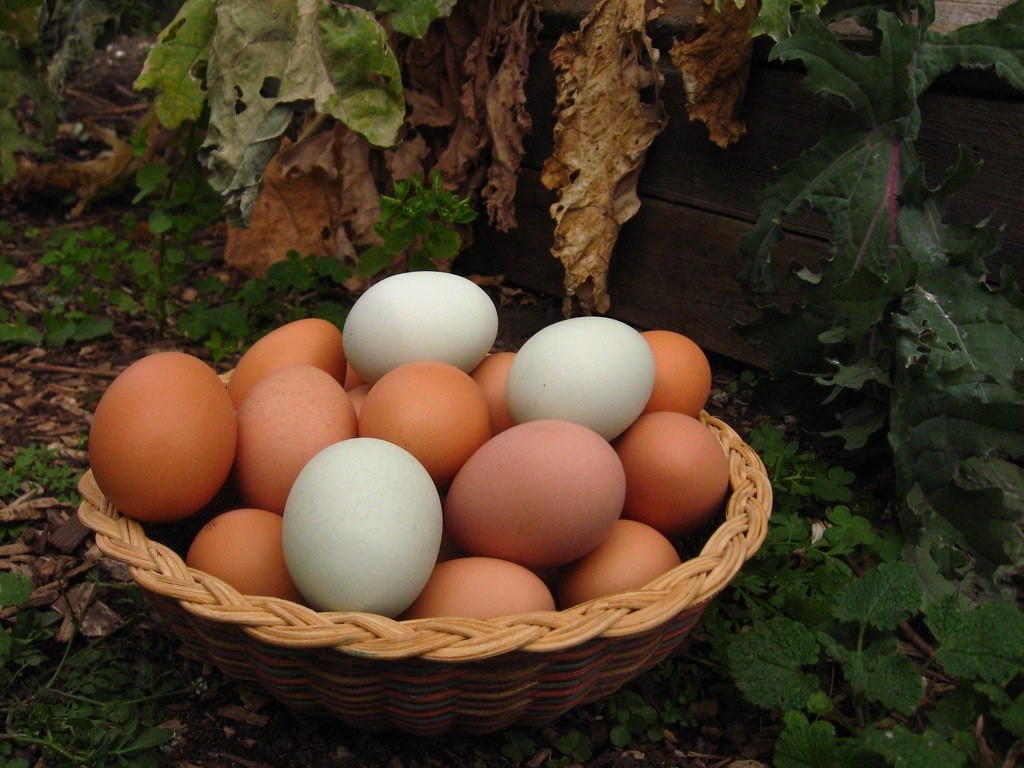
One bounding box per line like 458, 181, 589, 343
89, 270, 731, 619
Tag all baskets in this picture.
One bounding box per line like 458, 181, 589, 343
79, 365, 772, 740
